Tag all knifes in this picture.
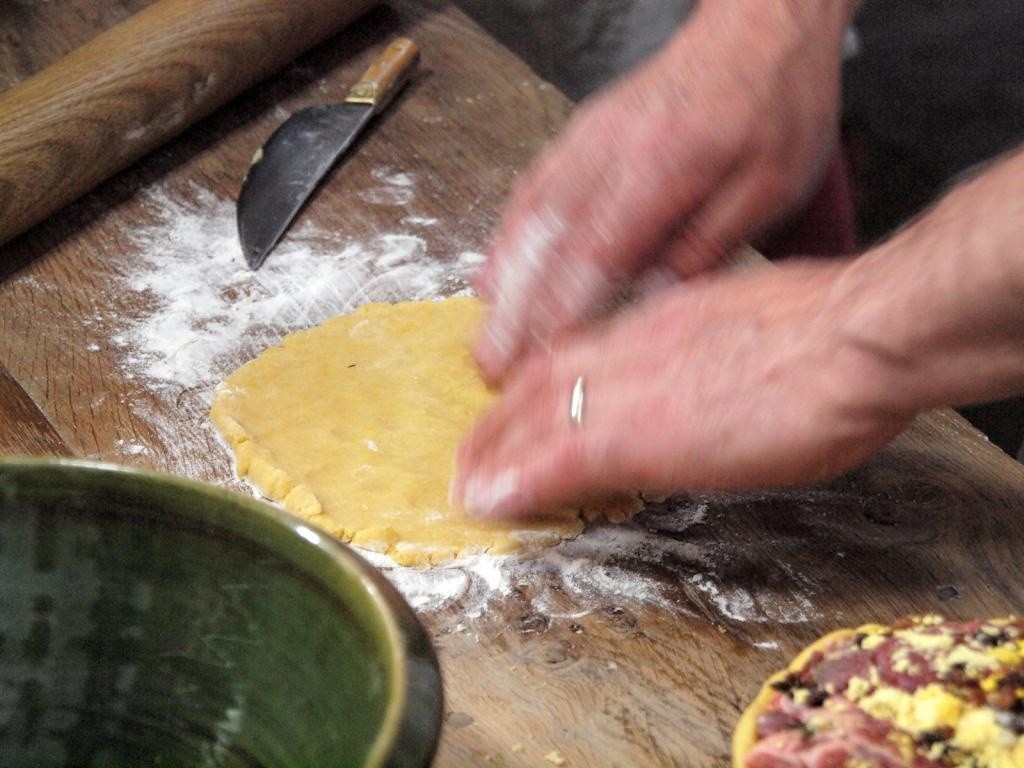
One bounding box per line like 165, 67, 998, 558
234, 36, 421, 270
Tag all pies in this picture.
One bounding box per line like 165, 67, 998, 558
731, 612, 1024, 768
212, 296, 670, 567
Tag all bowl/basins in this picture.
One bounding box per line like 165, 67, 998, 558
0, 456, 445, 768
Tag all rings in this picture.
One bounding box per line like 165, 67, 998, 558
571, 377, 587, 428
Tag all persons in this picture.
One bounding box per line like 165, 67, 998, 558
453, 2, 1024, 520
